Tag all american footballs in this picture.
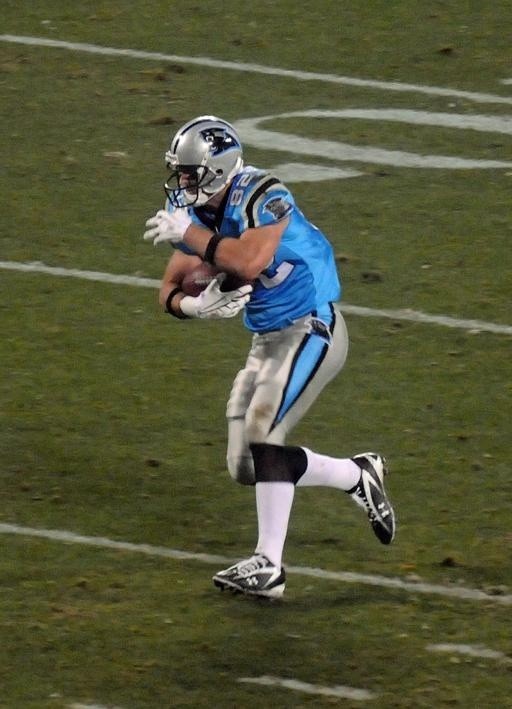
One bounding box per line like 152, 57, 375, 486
182, 261, 241, 297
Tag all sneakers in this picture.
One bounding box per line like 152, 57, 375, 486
347, 452, 394, 543
212, 555, 287, 599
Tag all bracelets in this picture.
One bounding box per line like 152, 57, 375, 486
162, 288, 191, 320
202, 233, 227, 266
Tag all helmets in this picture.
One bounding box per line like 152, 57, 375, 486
164, 116, 241, 206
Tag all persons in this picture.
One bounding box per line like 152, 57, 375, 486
143, 115, 397, 602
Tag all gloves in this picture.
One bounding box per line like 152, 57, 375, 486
180, 273, 252, 319
143, 202, 193, 245
